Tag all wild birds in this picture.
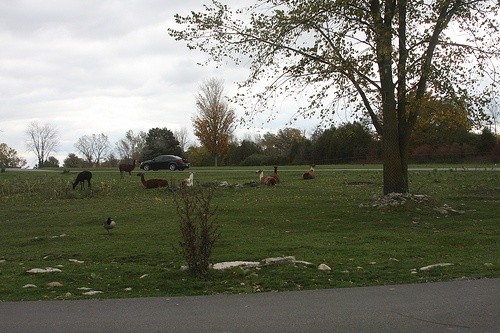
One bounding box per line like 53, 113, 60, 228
101, 216, 116, 234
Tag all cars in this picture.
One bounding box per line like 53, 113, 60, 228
140, 155, 189, 172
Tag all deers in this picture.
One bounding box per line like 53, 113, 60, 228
256, 169, 276, 185
137, 173, 169, 189
272, 166, 279, 185
181, 171, 196, 187
72, 171, 92, 190
118, 159, 138, 176
302, 163, 316, 180
